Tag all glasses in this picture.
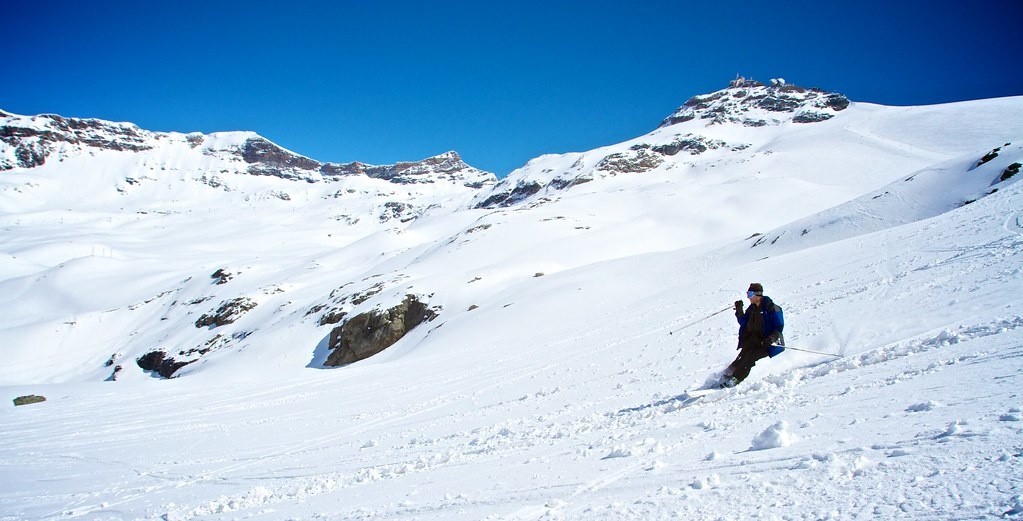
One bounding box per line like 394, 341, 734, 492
746, 291, 754, 298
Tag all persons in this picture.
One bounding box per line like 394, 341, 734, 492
712, 283, 786, 388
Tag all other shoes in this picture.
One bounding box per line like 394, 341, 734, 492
720, 376, 740, 388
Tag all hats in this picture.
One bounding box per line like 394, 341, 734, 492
748, 283, 763, 296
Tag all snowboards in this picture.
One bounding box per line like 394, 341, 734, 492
718, 363, 750, 388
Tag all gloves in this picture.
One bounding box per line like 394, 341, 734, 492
733, 300, 743, 313
762, 338, 772, 348
